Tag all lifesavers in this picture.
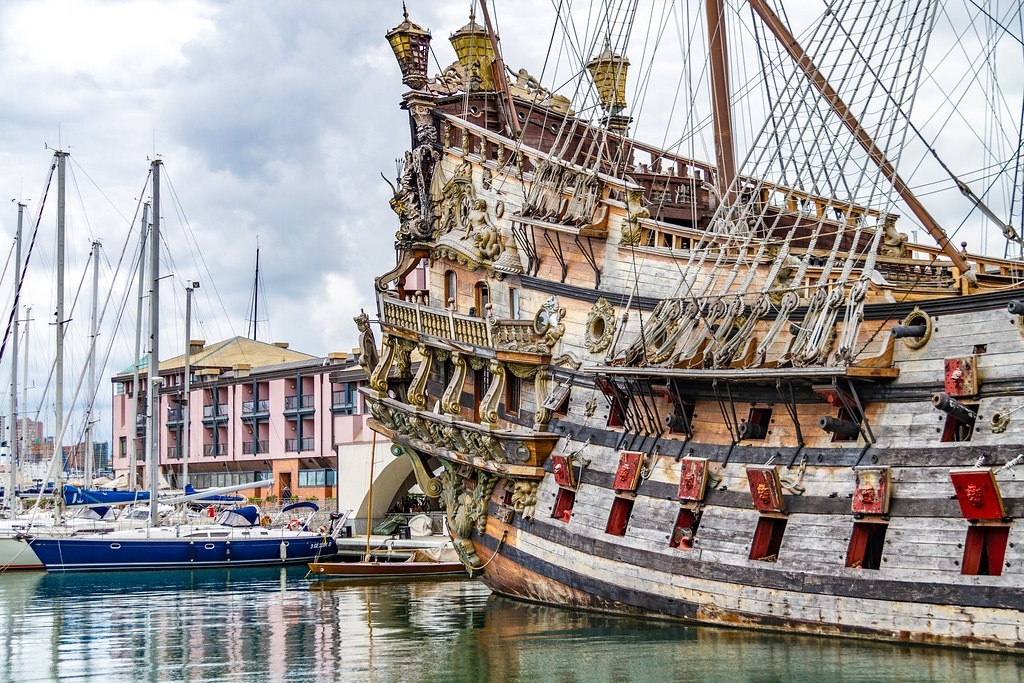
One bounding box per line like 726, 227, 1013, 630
288, 519, 300, 530
260, 515, 272, 525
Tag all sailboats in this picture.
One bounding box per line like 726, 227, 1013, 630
348, 0, 1024, 662
0, 145, 340, 572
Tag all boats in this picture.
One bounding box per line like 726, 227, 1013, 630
307, 495, 471, 574
